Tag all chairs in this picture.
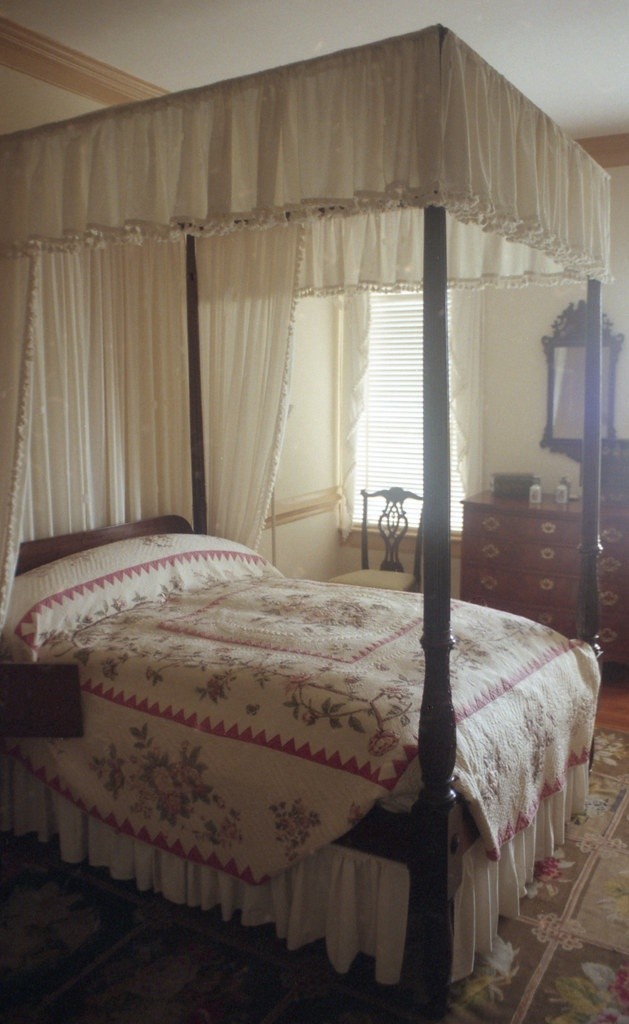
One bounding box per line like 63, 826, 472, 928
328, 487, 428, 592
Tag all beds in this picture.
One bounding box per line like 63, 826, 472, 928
0, 18, 610, 985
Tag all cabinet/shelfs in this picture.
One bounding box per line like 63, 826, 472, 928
455, 492, 628, 674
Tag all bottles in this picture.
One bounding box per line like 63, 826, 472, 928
556, 478, 568, 502
529, 475, 541, 504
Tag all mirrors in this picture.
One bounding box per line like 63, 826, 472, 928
540, 299, 625, 466
